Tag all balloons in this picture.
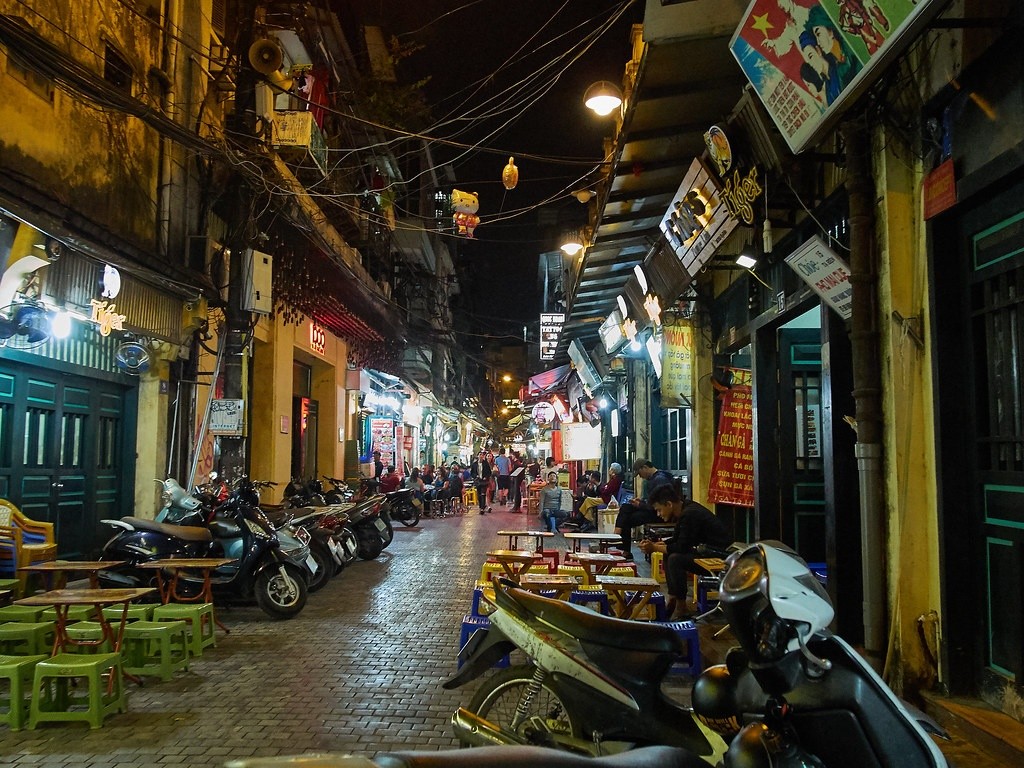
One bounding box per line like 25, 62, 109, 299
502, 157, 519, 189
451, 188, 480, 240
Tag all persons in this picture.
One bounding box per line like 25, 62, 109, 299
638, 483, 733, 620
568, 470, 604, 529
379, 456, 471, 518
577, 463, 622, 524
613, 458, 674, 561
373, 451, 383, 479
470, 448, 539, 514
540, 458, 569, 480
538, 471, 569, 532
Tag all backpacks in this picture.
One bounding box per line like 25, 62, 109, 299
648, 470, 685, 502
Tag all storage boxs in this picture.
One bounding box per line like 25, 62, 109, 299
598, 509, 636, 538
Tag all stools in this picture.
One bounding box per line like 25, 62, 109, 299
482, 562, 514, 582
38, 604, 103, 622
27, 653, 128, 731
543, 550, 559, 574
55, 620, 130, 654
693, 574, 720, 604
557, 564, 590, 584
431, 488, 477, 518
102, 603, 161, 621
0, 622, 55, 656
569, 590, 609, 616
474, 579, 493, 590
521, 497, 527, 508
565, 561, 582, 566
526, 565, 550, 574
653, 620, 704, 678
0, 654, 53, 733
697, 577, 721, 615
0, 604, 54, 622
625, 591, 666, 621
456, 614, 511, 670
148, 603, 218, 657
471, 589, 496, 616
651, 552, 667, 583
116, 620, 190, 683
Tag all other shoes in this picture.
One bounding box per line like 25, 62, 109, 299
672, 609, 694, 622
663, 597, 676, 612
500, 501, 503, 506
488, 508, 492, 513
480, 510, 484, 514
618, 553, 634, 563
580, 520, 591, 532
503, 496, 507, 505
599, 534, 623, 545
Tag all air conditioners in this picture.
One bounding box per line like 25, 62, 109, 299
376, 281, 392, 301
352, 247, 363, 265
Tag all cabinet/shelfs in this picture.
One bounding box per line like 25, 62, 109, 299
527, 485, 543, 515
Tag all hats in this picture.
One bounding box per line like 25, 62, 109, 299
632, 458, 649, 477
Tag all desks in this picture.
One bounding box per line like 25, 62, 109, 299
563, 532, 623, 554
464, 483, 472, 488
496, 530, 555, 562
486, 550, 543, 585
17, 560, 127, 619
596, 574, 660, 620
133, 559, 240, 635
463, 480, 475, 504
520, 573, 578, 599
12, 587, 158, 699
567, 553, 626, 585
692, 557, 725, 576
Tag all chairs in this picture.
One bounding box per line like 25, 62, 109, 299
0, 498, 59, 602
643, 523, 676, 561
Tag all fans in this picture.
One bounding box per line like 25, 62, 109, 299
0, 303, 55, 351
111, 341, 155, 377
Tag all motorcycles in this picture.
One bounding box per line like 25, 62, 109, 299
94, 465, 425, 622
440, 537, 955, 759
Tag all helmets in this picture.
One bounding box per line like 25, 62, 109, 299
692, 665, 743, 733
231, 487, 260, 508
325, 493, 344, 504
307, 479, 325, 492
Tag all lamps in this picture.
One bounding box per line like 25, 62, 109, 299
98, 264, 122, 301
571, 182, 597, 204
559, 233, 585, 256
610, 408, 622, 437
583, 81, 624, 117
732, 243, 761, 269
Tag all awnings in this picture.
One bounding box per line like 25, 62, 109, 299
528, 365, 573, 396
